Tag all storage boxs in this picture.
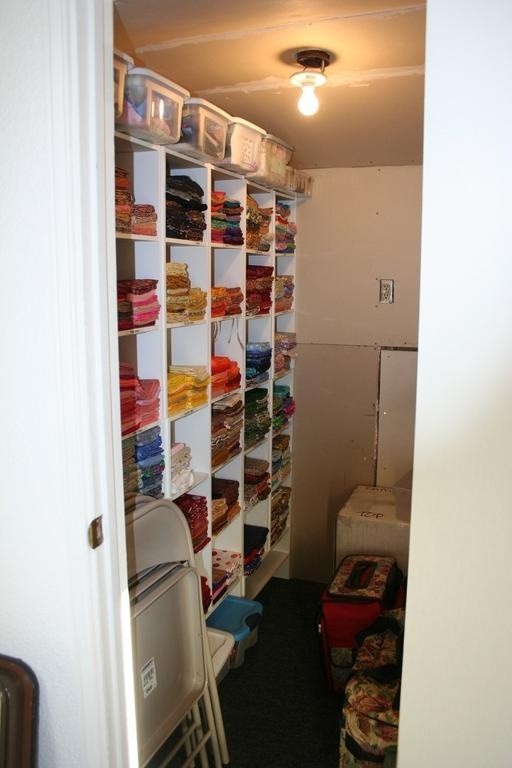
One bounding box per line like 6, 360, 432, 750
205, 595, 264, 687
335, 485, 411, 581
113, 46, 267, 175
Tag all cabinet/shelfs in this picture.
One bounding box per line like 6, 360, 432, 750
115, 130, 298, 624
